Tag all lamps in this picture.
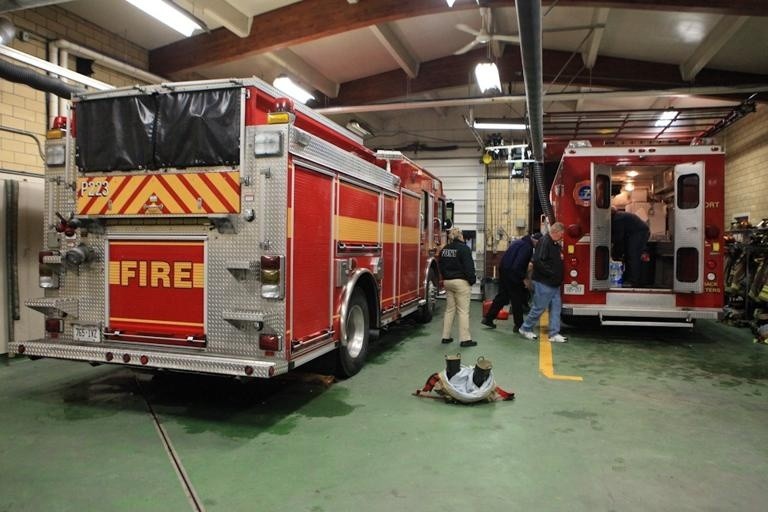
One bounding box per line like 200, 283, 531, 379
273, 74, 317, 106
127, 0, 211, 38
472, 118, 531, 130
475, 60, 503, 95
346, 119, 371, 138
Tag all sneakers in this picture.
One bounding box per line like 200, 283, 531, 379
459, 339, 478, 347
441, 337, 454, 344
480, 318, 498, 330
548, 332, 569, 344
512, 304, 537, 342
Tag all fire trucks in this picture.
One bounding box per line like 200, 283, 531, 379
538, 132, 728, 337
4, 66, 456, 385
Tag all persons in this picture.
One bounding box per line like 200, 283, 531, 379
481, 232, 543, 333
438, 227, 477, 347
610, 205, 658, 285
518, 221, 569, 343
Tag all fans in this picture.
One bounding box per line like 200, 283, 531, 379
453, 6, 520, 56
